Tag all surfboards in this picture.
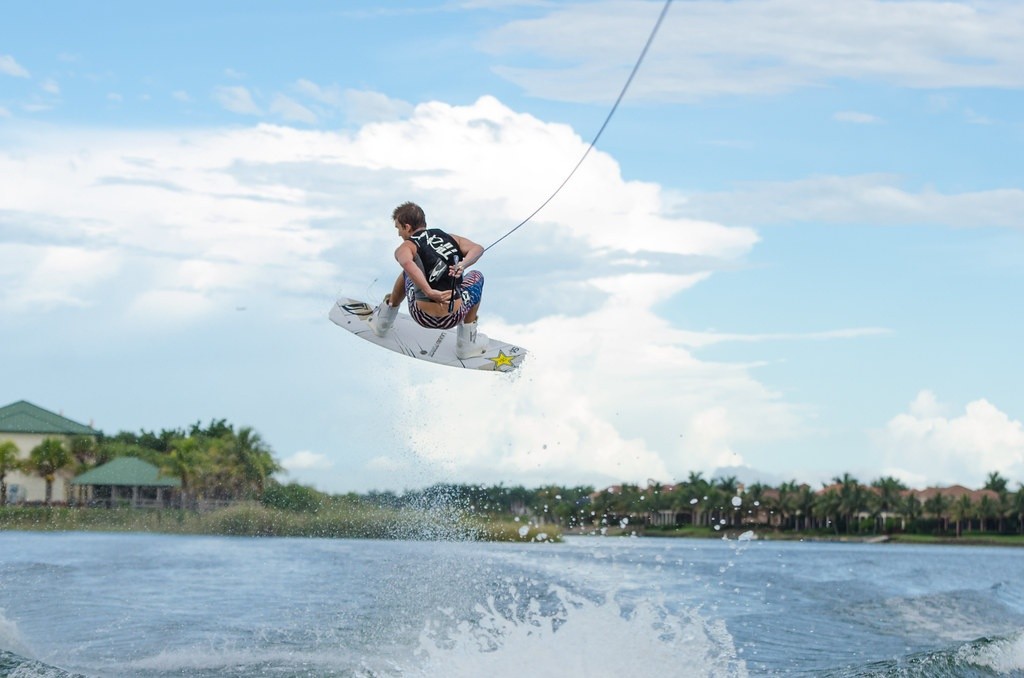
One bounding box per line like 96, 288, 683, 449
330, 296, 527, 372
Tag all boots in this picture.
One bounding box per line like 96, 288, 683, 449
368, 294, 401, 337
455, 317, 488, 359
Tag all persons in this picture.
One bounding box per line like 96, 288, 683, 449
367, 201, 489, 359
